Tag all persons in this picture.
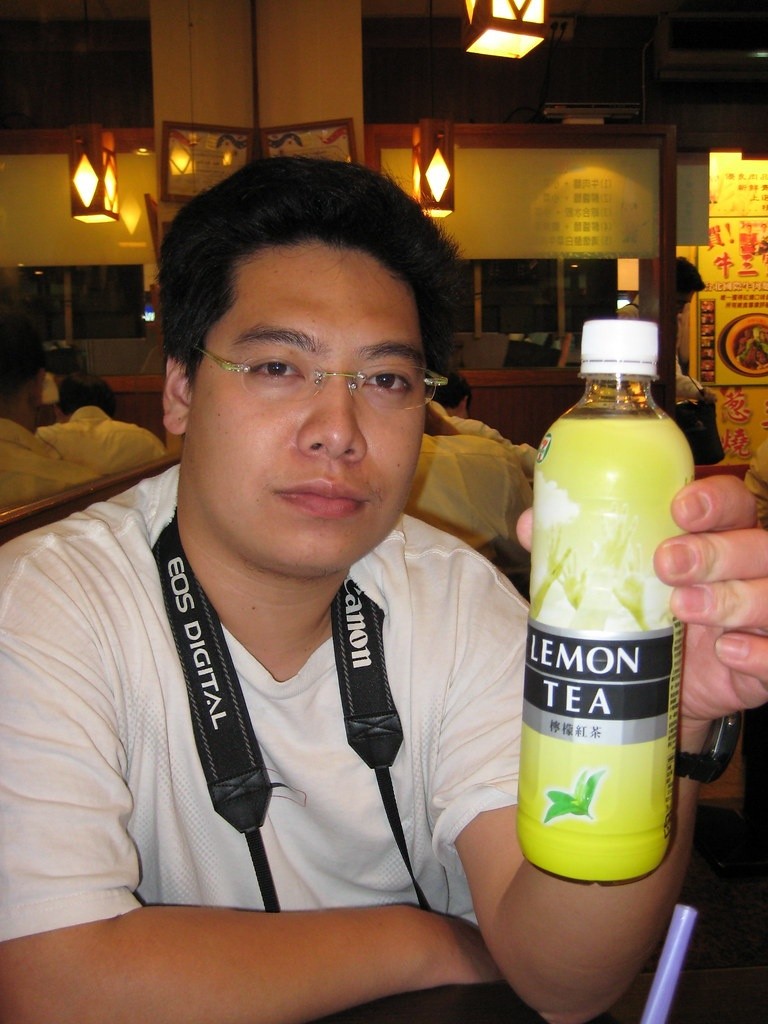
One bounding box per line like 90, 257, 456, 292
0, 158, 768, 1024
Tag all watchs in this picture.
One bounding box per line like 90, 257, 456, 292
674, 712, 741, 783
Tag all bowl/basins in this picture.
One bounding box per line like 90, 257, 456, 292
725, 318, 768, 373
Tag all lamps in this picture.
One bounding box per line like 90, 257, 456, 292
65, 129, 122, 223
459, 0, 550, 64
414, 119, 456, 217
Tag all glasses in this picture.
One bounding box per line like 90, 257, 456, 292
193, 347, 448, 410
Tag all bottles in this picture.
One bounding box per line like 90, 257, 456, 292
517, 320, 696, 883
